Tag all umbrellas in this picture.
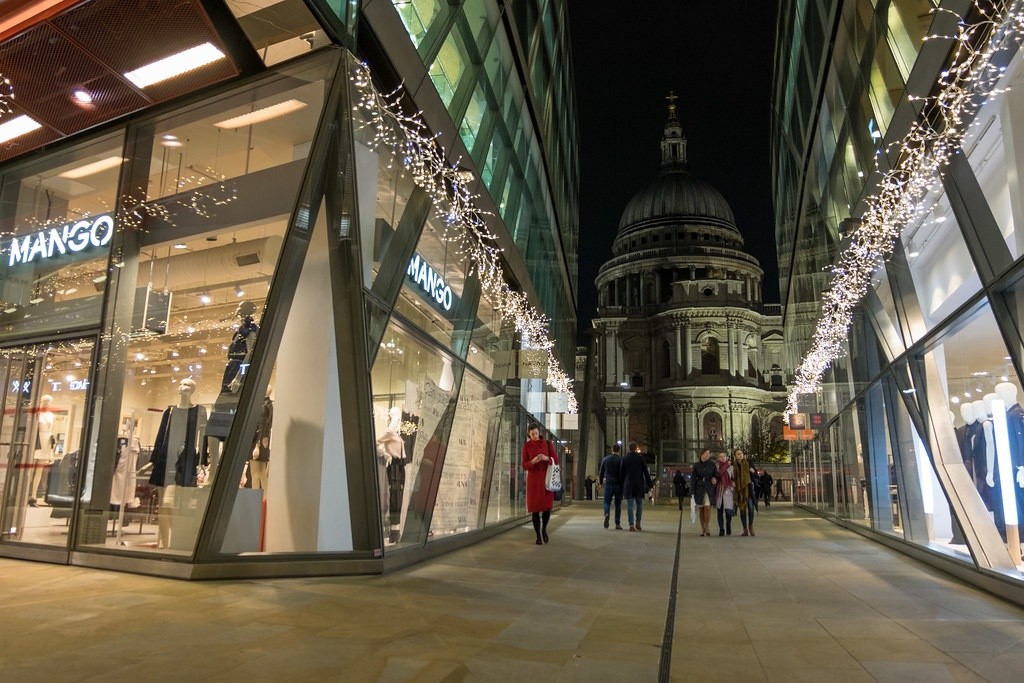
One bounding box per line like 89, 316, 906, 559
748, 481, 758, 512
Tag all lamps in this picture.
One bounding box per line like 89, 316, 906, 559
266, 278, 271, 289
932, 202, 947, 223
46, 361, 54, 370
172, 348, 179, 357
171, 375, 177, 383
91, 275, 106, 292
439, 355, 457, 392
141, 378, 147, 386
150, 366, 156, 374
74, 357, 82, 367
907, 237, 919, 257
174, 363, 180, 371
233, 285, 244, 297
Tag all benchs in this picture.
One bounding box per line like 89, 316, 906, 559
43, 448, 198, 537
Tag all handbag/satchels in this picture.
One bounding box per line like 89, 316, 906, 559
545, 458, 562, 492
691, 495, 697, 524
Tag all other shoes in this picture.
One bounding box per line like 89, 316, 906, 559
720, 529, 724, 536
749, 525, 755, 536
604, 514, 610, 528
742, 529, 747, 536
630, 527, 635, 532
536, 538, 541, 545
542, 529, 549, 543
727, 526, 730, 536
637, 524, 641, 530
700, 530, 704, 536
616, 524, 623, 529
705, 528, 710, 536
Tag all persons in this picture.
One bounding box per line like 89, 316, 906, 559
732, 448, 758, 537
760, 470, 774, 508
673, 470, 686, 512
621, 442, 652, 532
600, 444, 623, 530
521, 423, 560, 545
28, 395, 55, 501
377, 408, 408, 532
193, 300, 259, 486
249, 385, 273, 502
775, 476, 788, 503
108, 419, 139, 534
948, 382, 1024, 561
716, 450, 735, 537
585, 475, 599, 501
148, 378, 207, 551
690, 448, 720, 537
